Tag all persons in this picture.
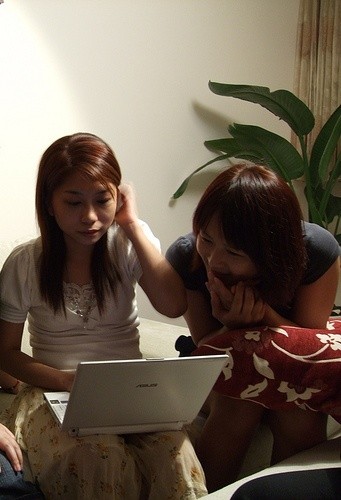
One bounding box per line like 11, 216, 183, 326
0, 133, 209, 500
166, 163, 341, 495
0, 423, 23, 472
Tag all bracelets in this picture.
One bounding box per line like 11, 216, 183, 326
2, 381, 19, 390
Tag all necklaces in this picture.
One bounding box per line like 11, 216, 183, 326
66, 263, 93, 330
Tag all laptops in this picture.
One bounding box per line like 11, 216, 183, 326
43, 354, 230, 436
